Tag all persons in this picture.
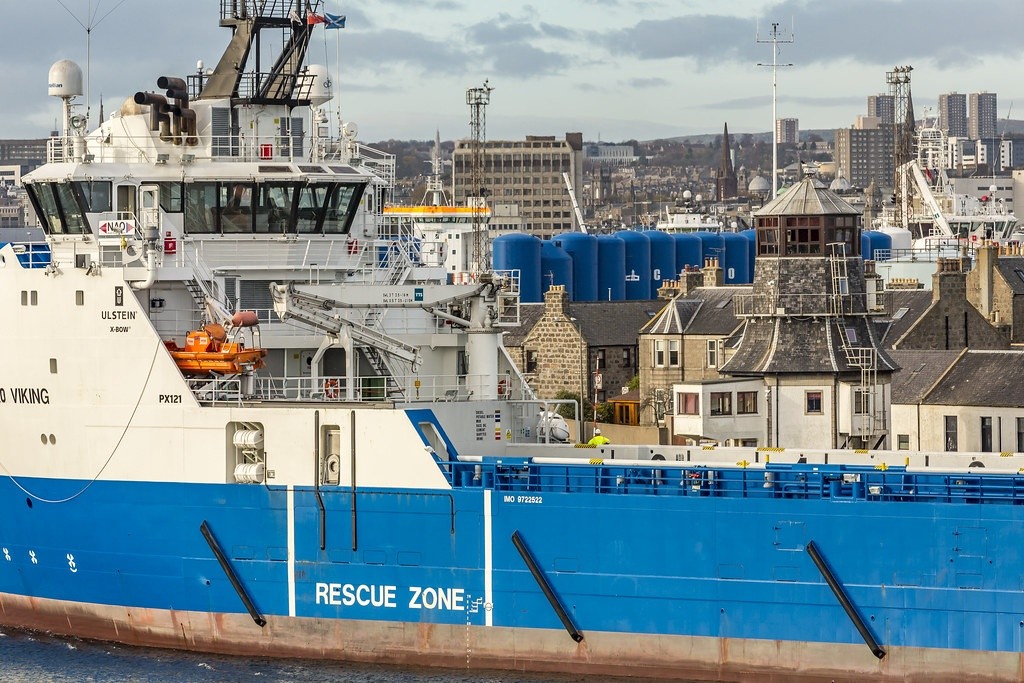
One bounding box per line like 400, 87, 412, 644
588, 429, 609, 445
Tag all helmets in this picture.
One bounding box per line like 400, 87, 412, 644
595, 429, 601, 433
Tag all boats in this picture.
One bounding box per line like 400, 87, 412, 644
165, 322, 265, 375
870, 106, 1024, 289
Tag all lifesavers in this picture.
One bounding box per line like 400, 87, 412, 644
325, 381, 339, 399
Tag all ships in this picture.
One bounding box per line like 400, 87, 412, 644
0, 0, 1024, 683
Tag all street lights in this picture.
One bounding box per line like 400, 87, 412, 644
553, 312, 585, 443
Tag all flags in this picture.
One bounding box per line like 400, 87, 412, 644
325, 14, 345, 30
290, 11, 303, 26
307, 10, 324, 24
